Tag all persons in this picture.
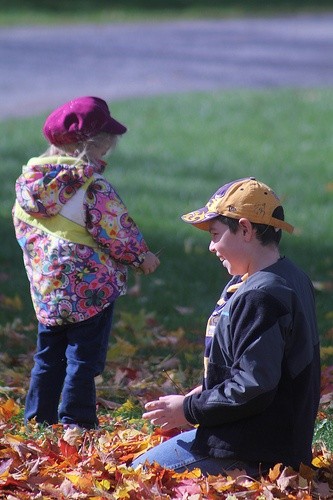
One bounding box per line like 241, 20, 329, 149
10, 95, 160, 433
125, 176, 322, 482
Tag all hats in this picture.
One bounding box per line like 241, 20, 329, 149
180, 176, 295, 234
43, 96, 126, 146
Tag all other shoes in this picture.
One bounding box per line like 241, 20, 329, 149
63, 423, 104, 434
